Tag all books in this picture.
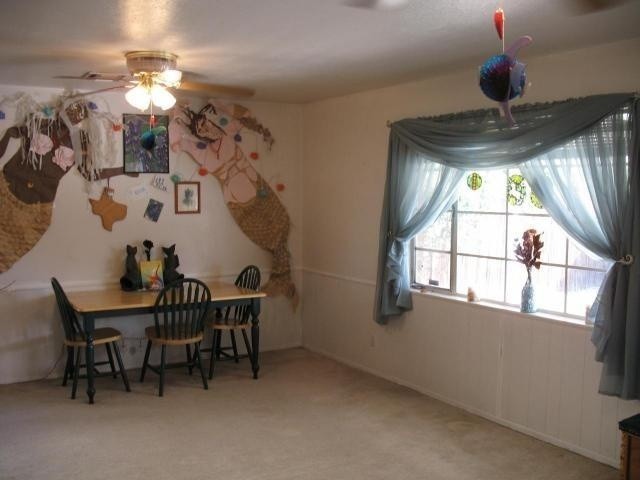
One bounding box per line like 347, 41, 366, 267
137, 259, 165, 293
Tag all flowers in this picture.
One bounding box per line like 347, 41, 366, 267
512, 228, 545, 269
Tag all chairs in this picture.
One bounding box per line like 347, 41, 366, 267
51, 277, 132, 399
189, 264, 261, 380
140, 279, 211, 396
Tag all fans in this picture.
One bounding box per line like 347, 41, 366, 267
65, 49, 255, 101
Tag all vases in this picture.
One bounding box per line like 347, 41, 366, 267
520, 266, 535, 313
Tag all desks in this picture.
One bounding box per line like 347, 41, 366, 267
59, 280, 268, 405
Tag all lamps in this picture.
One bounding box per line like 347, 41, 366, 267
124, 68, 183, 111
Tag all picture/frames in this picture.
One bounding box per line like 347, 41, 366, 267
174, 181, 200, 214
123, 114, 170, 174
